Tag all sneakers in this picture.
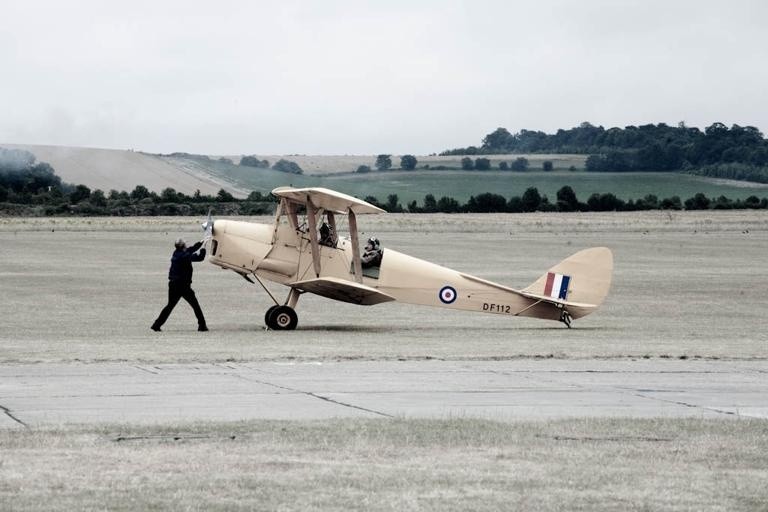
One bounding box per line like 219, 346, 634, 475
198, 328, 209, 331
151, 325, 161, 332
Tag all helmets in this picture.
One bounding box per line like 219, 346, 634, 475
366, 237, 380, 252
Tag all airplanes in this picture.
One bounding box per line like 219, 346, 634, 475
191, 182, 623, 334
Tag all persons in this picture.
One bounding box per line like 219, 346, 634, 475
360, 236, 381, 269
150, 237, 210, 331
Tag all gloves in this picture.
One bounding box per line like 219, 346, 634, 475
201, 236, 210, 248
